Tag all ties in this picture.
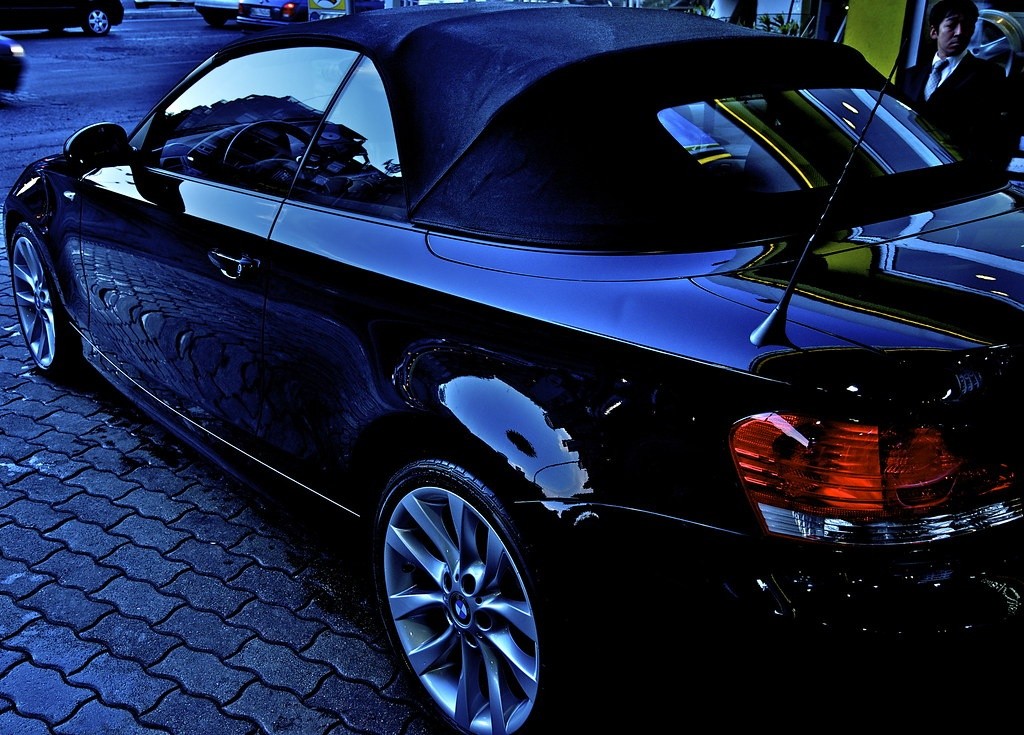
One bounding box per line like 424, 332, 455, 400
924, 59, 949, 101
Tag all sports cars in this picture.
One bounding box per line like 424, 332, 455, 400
3, 2, 1023, 734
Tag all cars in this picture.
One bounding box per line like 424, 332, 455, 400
0, 0, 125, 36
236, 0, 385, 29
1, 34, 27, 92
193, 0, 240, 25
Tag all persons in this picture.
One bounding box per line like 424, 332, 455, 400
895, 0, 1019, 178
